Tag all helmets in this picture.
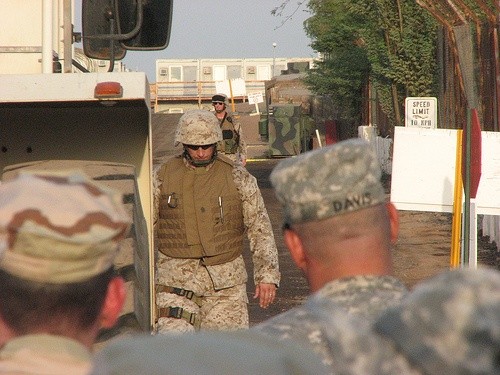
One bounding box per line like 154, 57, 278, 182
212, 93, 229, 107
173, 109, 224, 147
377, 266, 500, 375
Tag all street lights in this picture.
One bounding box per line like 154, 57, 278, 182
273, 42, 277, 77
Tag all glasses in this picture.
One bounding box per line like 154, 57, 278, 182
214, 102, 224, 105
182, 143, 217, 150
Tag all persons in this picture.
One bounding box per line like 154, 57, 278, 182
0, 170, 130, 375
152, 112, 280, 335
93, 137, 500, 375
212, 92, 247, 166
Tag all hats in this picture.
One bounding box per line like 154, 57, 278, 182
268, 137, 386, 233
0, 167, 134, 284
94, 327, 332, 375
